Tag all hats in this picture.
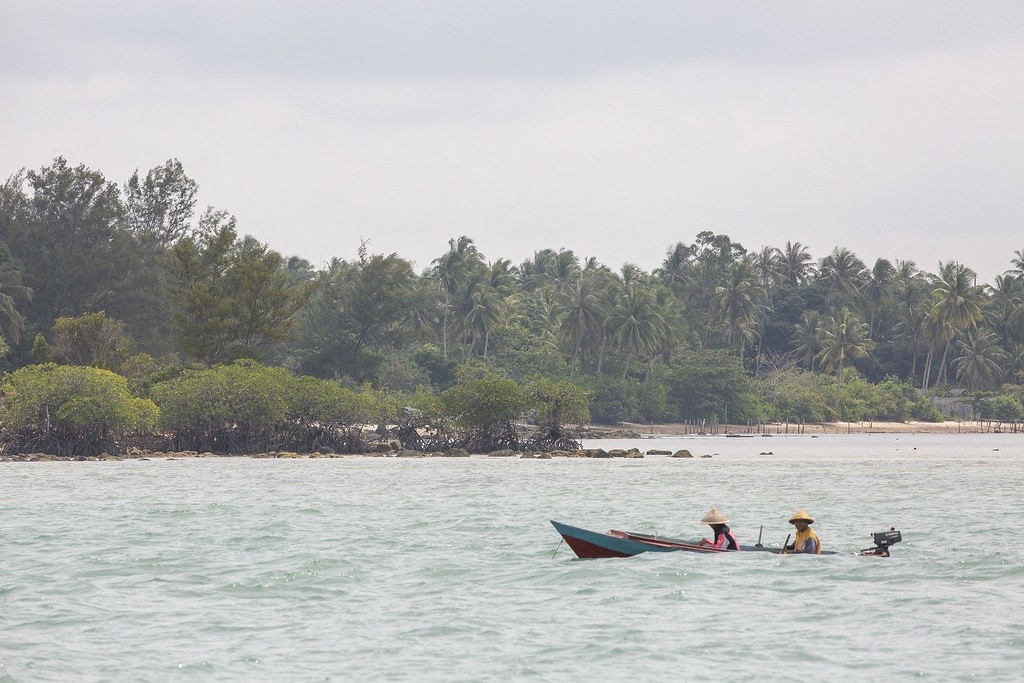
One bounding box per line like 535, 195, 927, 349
701, 508, 729, 524
788, 510, 814, 524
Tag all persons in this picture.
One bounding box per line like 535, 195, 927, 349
699, 508, 741, 551
778, 511, 821, 555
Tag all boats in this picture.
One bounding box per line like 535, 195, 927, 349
549, 517, 905, 563
